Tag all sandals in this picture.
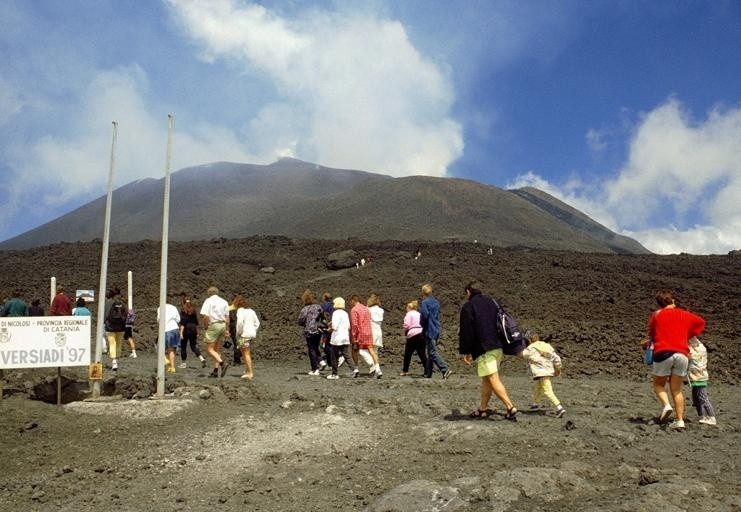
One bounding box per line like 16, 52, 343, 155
470, 406, 566, 419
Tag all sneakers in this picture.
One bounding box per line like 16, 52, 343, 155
165, 359, 186, 373
129, 352, 137, 358
699, 415, 716, 424
308, 355, 382, 380
443, 370, 452, 379
202, 359, 206, 368
221, 362, 229, 377
208, 372, 218, 378
660, 405, 685, 429
112, 359, 117, 369
241, 374, 254, 378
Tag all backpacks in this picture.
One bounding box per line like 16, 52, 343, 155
108, 301, 126, 323
491, 298, 521, 344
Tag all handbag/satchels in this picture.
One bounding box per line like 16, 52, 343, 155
645, 349, 653, 365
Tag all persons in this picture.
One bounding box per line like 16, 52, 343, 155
228, 297, 261, 381
459, 280, 515, 420
400, 300, 427, 376
200, 287, 230, 378
299, 289, 385, 380
521, 330, 565, 419
152, 295, 182, 374
101, 287, 137, 371
1, 283, 93, 316
688, 337, 717, 425
177, 297, 208, 369
641, 289, 705, 429
419, 284, 452, 379
